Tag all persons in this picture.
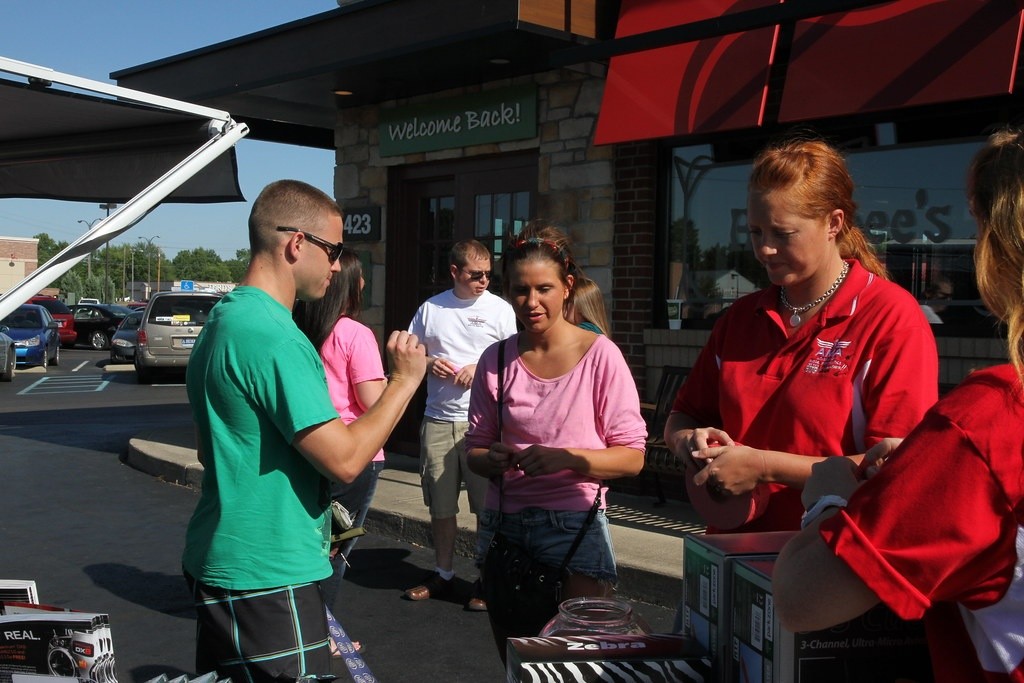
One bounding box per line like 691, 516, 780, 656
187, 179, 427, 683
664, 141, 938, 534
562, 277, 609, 334
464, 218, 648, 673
770, 126, 1024, 683
292, 248, 392, 658
403, 238, 516, 608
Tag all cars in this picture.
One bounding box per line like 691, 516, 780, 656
127, 291, 225, 380
0, 303, 61, 369
65, 304, 134, 351
110, 311, 145, 364
126, 302, 147, 313
24, 295, 79, 349
0, 324, 18, 381
77, 297, 100, 303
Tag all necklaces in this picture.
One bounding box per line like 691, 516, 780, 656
780, 260, 849, 327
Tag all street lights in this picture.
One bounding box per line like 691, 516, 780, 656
129, 248, 137, 299
99, 203, 117, 303
77, 217, 104, 281
138, 234, 160, 300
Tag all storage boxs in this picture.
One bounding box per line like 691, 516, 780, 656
731, 559, 936, 683
682, 531, 800, 683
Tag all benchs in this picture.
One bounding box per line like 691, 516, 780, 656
642, 365, 694, 507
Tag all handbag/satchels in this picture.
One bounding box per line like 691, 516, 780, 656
482, 532, 566, 633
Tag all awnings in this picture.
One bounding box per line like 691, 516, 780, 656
0, 56, 250, 325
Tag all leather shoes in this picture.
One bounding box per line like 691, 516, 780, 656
469, 576, 487, 611
405, 572, 456, 600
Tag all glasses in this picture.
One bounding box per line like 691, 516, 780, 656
277, 225, 344, 261
456, 264, 492, 280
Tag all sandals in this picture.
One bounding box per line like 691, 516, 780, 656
328, 638, 362, 659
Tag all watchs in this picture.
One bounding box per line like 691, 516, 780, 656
800, 494, 848, 531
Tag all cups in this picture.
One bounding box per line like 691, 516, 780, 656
667, 298, 684, 330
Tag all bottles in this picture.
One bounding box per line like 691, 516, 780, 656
536, 596, 656, 638
